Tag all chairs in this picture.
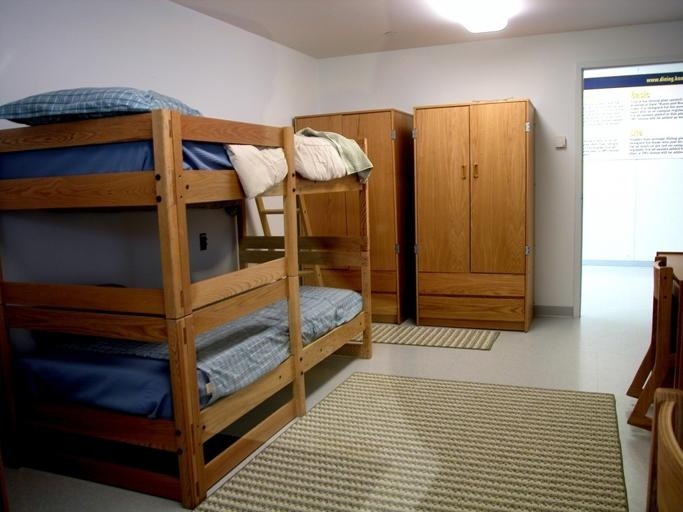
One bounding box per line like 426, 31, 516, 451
645, 387, 683, 512
626, 256, 675, 431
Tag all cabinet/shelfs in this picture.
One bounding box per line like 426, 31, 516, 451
295, 108, 417, 325
414, 99, 535, 332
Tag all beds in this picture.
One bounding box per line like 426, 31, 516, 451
0, 109, 372, 509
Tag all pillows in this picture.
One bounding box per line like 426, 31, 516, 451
0, 86, 203, 127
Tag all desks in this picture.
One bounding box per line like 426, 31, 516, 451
656, 251, 683, 389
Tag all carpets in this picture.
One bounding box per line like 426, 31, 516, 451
191, 372, 630, 512
349, 323, 501, 351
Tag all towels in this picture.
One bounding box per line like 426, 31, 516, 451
296, 126, 373, 185
